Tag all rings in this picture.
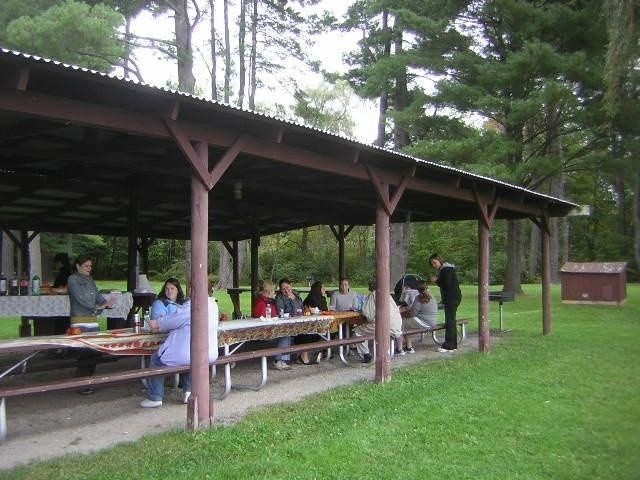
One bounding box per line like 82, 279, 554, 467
151, 324, 152, 325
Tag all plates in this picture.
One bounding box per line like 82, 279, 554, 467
322, 310, 335, 314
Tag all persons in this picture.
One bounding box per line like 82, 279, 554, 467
303, 281, 335, 359
330, 278, 359, 350
141, 278, 219, 408
151, 278, 185, 320
275, 279, 312, 365
395, 280, 437, 355
47, 253, 74, 359
252, 279, 290, 370
429, 254, 462, 353
67, 255, 113, 395
354, 280, 402, 363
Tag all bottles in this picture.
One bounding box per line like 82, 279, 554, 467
358, 299, 363, 312
144, 310, 151, 329
279, 309, 284, 318
32, 271, 40, 296
352, 297, 358, 312
67, 328, 81, 335
19, 272, 29, 295
0, 272, 7, 296
7, 270, 19, 296
265, 304, 271, 318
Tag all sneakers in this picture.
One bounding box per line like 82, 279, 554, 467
402, 346, 415, 354
183, 393, 190, 403
273, 344, 372, 370
141, 398, 162, 408
438, 346, 454, 352
393, 348, 405, 356
78, 388, 94, 394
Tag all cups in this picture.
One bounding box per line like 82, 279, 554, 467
297, 309, 302, 315
329, 305, 335, 312
283, 312, 290, 320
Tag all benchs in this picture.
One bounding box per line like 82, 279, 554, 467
0, 318, 470, 435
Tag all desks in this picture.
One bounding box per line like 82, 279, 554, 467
0, 293, 156, 329
